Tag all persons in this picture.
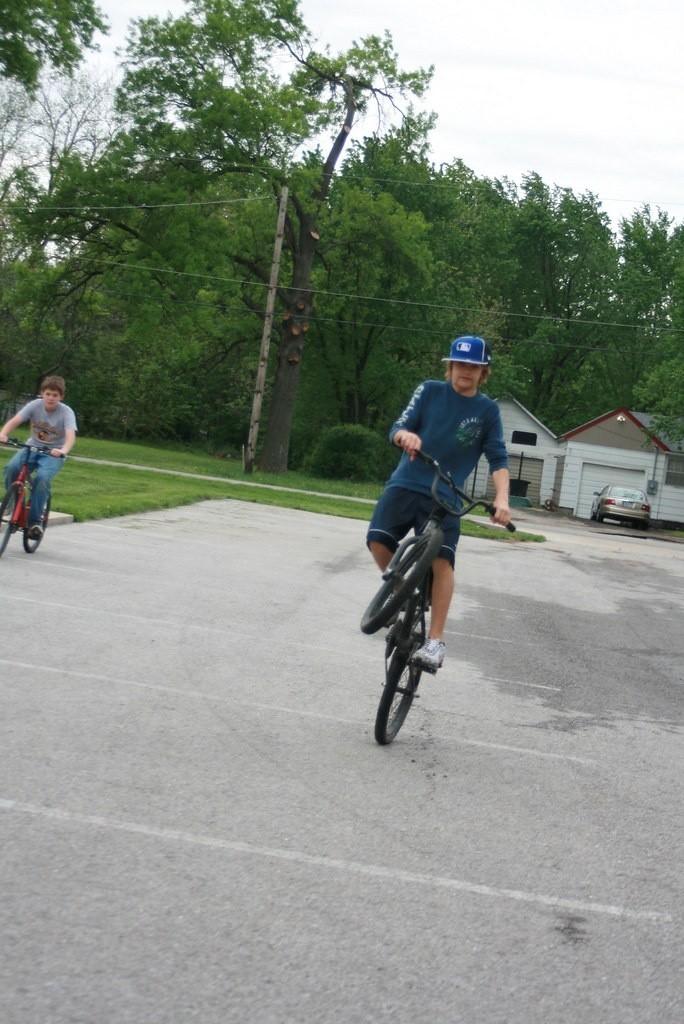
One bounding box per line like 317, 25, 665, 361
0, 376, 77, 540
366, 336, 511, 671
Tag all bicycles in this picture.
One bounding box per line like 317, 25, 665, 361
360, 437, 516, 745
0, 438, 65, 557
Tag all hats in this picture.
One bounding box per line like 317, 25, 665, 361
440, 335, 493, 366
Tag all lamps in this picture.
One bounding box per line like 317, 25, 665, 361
617, 415, 626, 422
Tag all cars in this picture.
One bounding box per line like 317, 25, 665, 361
590, 485, 651, 530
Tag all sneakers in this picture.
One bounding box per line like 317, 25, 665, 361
3, 514, 11, 521
411, 636, 447, 671
28, 524, 44, 540
384, 594, 403, 625
